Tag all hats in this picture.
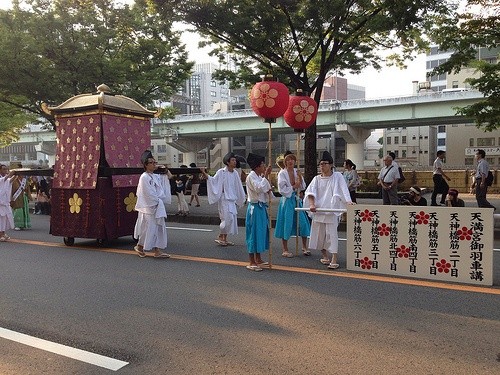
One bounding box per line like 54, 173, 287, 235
448, 188, 458, 197
222, 152, 241, 168
246, 153, 266, 170
141, 150, 153, 168
321, 151, 333, 164
284, 151, 294, 159
411, 186, 422, 196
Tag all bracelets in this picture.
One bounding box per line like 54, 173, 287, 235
390, 185, 392, 188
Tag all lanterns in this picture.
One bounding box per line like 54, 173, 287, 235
251, 81, 318, 132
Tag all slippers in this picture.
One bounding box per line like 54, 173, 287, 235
154, 252, 170, 258
257, 261, 270, 267
214, 239, 227, 245
247, 264, 262, 272
134, 246, 146, 258
282, 248, 340, 269
226, 240, 235, 246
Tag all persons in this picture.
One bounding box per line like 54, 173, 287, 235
0, 164, 54, 241
132, 149, 496, 271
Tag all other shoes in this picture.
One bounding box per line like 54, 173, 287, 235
188, 203, 191, 206
195, 205, 200, 207
0, 234, 10, 241
14, 227, 21, 230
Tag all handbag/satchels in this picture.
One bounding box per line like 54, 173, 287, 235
397, 167, 405, 184
346, 171, 360, 188
487, 171, 493, 187
377, 182, 383, 197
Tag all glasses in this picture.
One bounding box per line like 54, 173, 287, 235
318, 162, 331, 166
147, 161, 156, 164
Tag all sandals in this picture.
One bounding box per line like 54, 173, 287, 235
183, 211, 191, 217
176, 211, 181, 216
